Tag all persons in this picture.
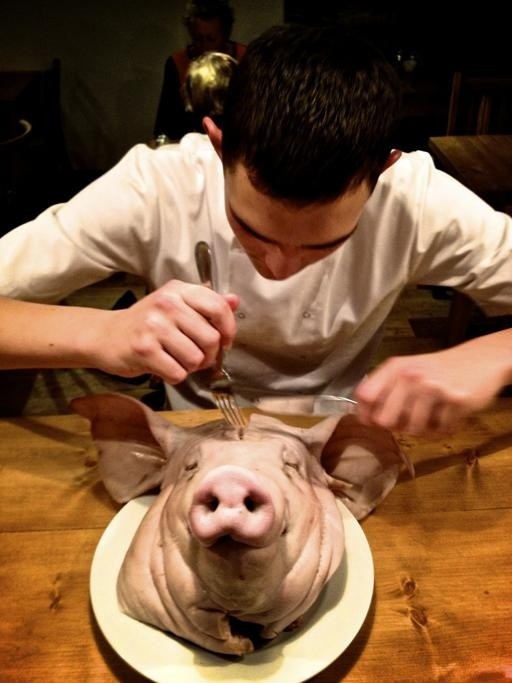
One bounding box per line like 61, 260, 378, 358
2, 18, 511, 438
152, 0, 252, 145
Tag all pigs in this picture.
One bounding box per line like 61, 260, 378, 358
69, 386, 404, 658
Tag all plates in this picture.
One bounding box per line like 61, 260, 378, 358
89, 486, 374, 683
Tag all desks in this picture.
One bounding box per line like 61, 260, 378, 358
0, 397, 512, 683
427, 133, 512, 347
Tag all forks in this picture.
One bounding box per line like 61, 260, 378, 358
196, 239, 245, 429
